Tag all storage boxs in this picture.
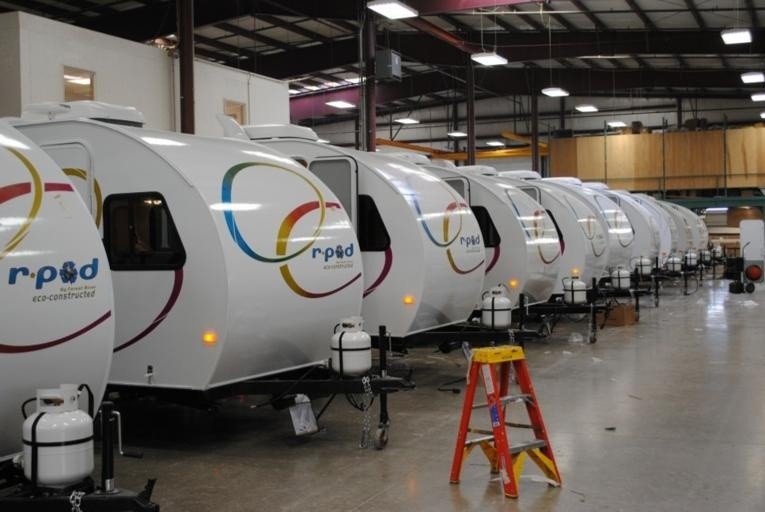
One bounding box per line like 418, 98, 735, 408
598, 303, 636, 326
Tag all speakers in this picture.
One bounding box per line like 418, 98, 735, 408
373, 49, 403, 80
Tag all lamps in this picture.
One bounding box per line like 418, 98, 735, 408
575, 37, 599, 113
367, 0, 419, 20
541, 17, 569, 98
607, 65, 627, 128
741, 72, 765, 119
471, 6, 508, 66
325, 100, 505, 147
721, 0, 752, 45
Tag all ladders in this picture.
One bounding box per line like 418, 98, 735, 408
450, 345, 562, 498
